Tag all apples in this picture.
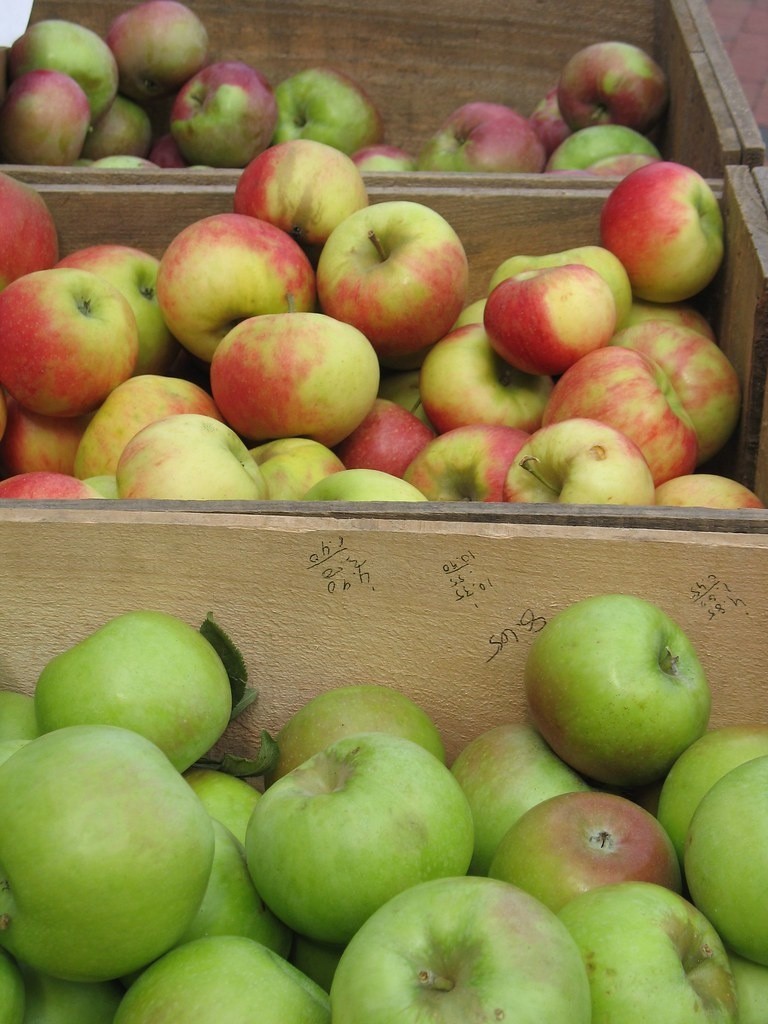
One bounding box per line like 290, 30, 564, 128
1, 592, 768, 1024
1, 140, 763, 510
6, 0, 669, 169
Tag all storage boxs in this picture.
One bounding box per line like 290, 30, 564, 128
0, 0, 768, 799
